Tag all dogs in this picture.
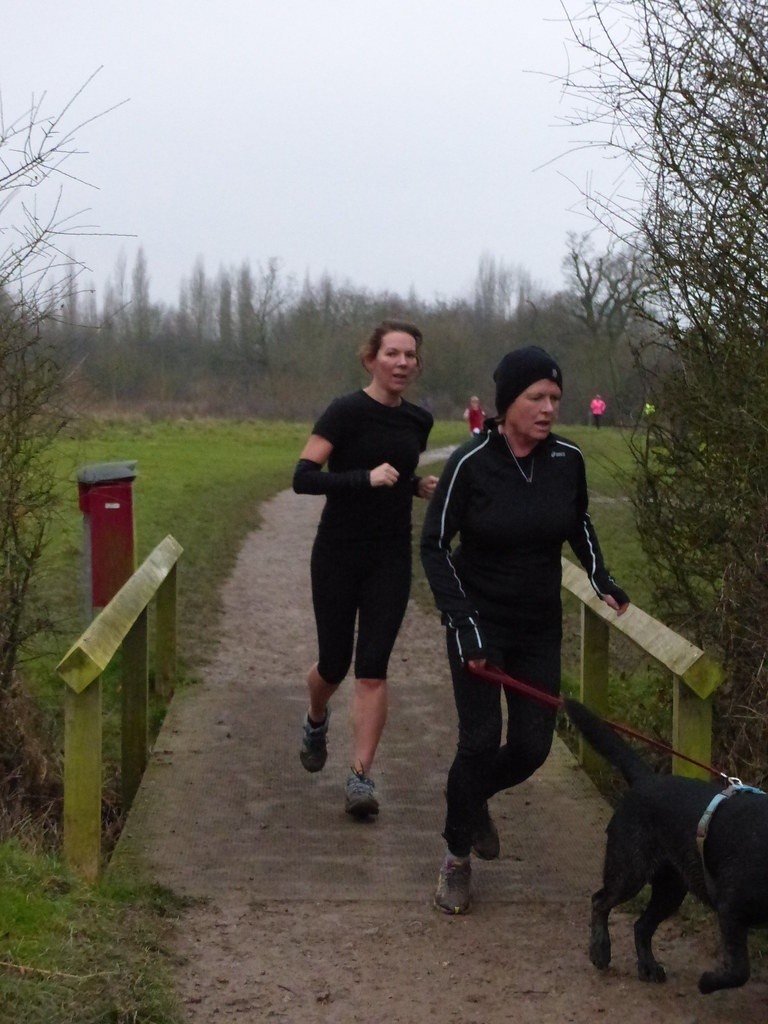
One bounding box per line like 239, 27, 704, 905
562, 697, 768, 994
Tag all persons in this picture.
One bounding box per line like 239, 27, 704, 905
590, 395, 606, 430
420, 345, 629, 917
293, 320, 440, 812
465, 396, 485, 437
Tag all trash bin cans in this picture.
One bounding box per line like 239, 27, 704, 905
76, 460, 137, 604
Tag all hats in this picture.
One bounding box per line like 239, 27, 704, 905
493, 345, 561, 415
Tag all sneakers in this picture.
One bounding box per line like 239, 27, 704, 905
344, 758, 380, 815
299, 702, 332, 773
433, 858, 472, 915
446, 790, 501, 860
